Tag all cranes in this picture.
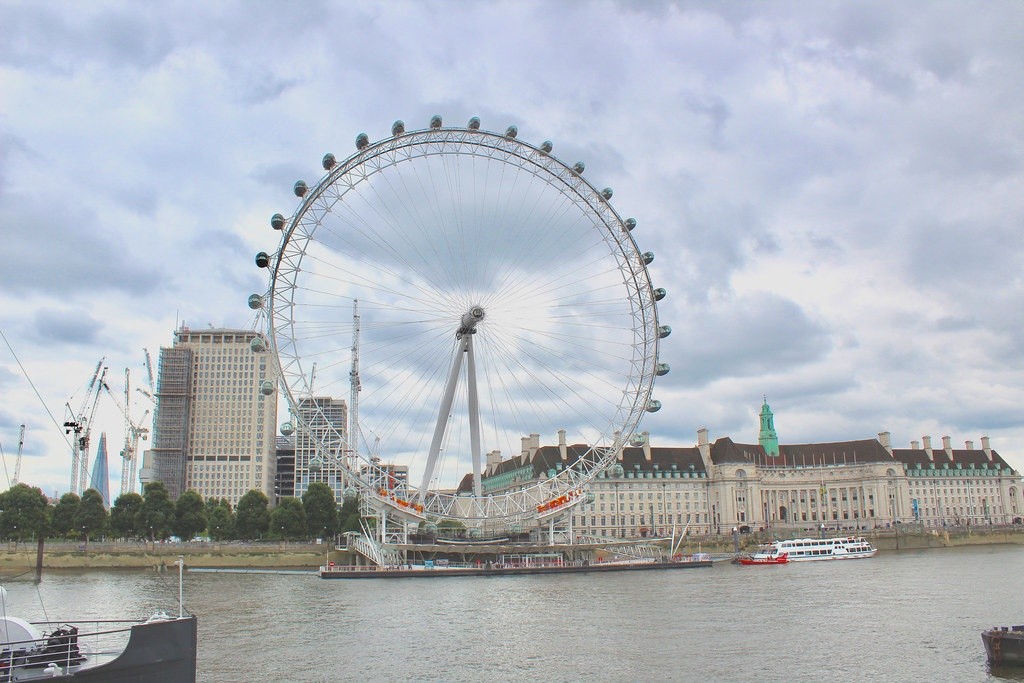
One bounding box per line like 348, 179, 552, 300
11, 421, 26, 488
60, 345, 160, 502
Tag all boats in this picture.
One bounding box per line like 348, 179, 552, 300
753, 534, 878, 561
736, 552, 790, 565
0, 556, 198, 683
